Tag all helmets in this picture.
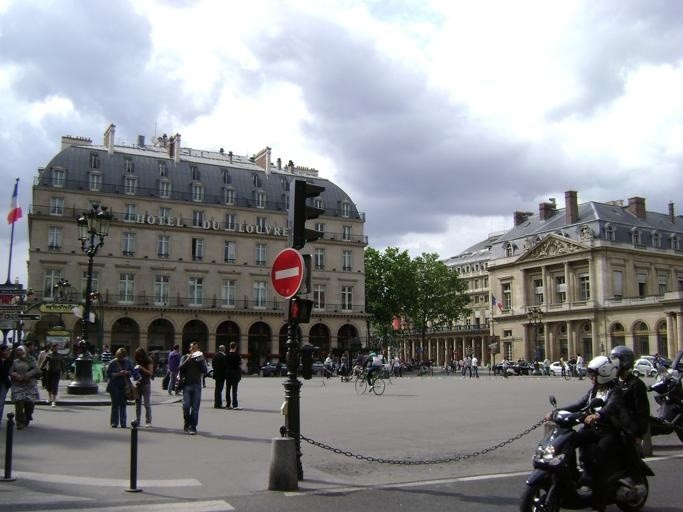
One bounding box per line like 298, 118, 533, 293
610, 345, 635, 372
586, 355, 617, 384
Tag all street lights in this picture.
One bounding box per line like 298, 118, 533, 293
526, 307, 544, 375
67, 202, 113, 395
54, 277, 71, 330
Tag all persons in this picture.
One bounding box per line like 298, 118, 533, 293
606, 345, 649, 488
307, 346, 479, 380
652, 353, 658, 368
542, 355, 621, 498
498, 355, 551, 377
576, 351, 583, 380
558, 354, 566, 378
567, 355, 576, 377
0, 331, 242, 435
362, 350, 381, 392
27, 288, 33, 300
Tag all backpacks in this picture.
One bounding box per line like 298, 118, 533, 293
372, 356, 382, 367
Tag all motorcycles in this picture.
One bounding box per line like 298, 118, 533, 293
518, 366, 683, 512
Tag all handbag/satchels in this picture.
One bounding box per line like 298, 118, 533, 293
124, 383, 140, 400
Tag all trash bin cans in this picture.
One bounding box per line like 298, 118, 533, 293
381, 364, 390, 379
281, 363, 288, 376
91, 361, 104, 383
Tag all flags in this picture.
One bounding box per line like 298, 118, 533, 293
5, 181, 22, 225
491, 295, 503, 311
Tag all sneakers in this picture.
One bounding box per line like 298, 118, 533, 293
112, 420, 152, 429
184, 422, 196, 434
576, 485, 593, 497
214, 406, 244, 410
16, 419, 29, 430
369, 386, 374, 392
46, 399, 56, 407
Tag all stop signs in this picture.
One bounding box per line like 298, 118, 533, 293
269, 247, 305, 299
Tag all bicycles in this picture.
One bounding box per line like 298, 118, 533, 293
355, 366, 386, 396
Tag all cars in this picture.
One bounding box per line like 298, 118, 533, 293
632, 355, 673, 378
206, 357, 214, 378
257, 358, 324, 377
492, 355, 586, 378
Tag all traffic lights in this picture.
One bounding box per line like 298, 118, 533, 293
289, 298, 315, 323
287, 179, 327, 249
